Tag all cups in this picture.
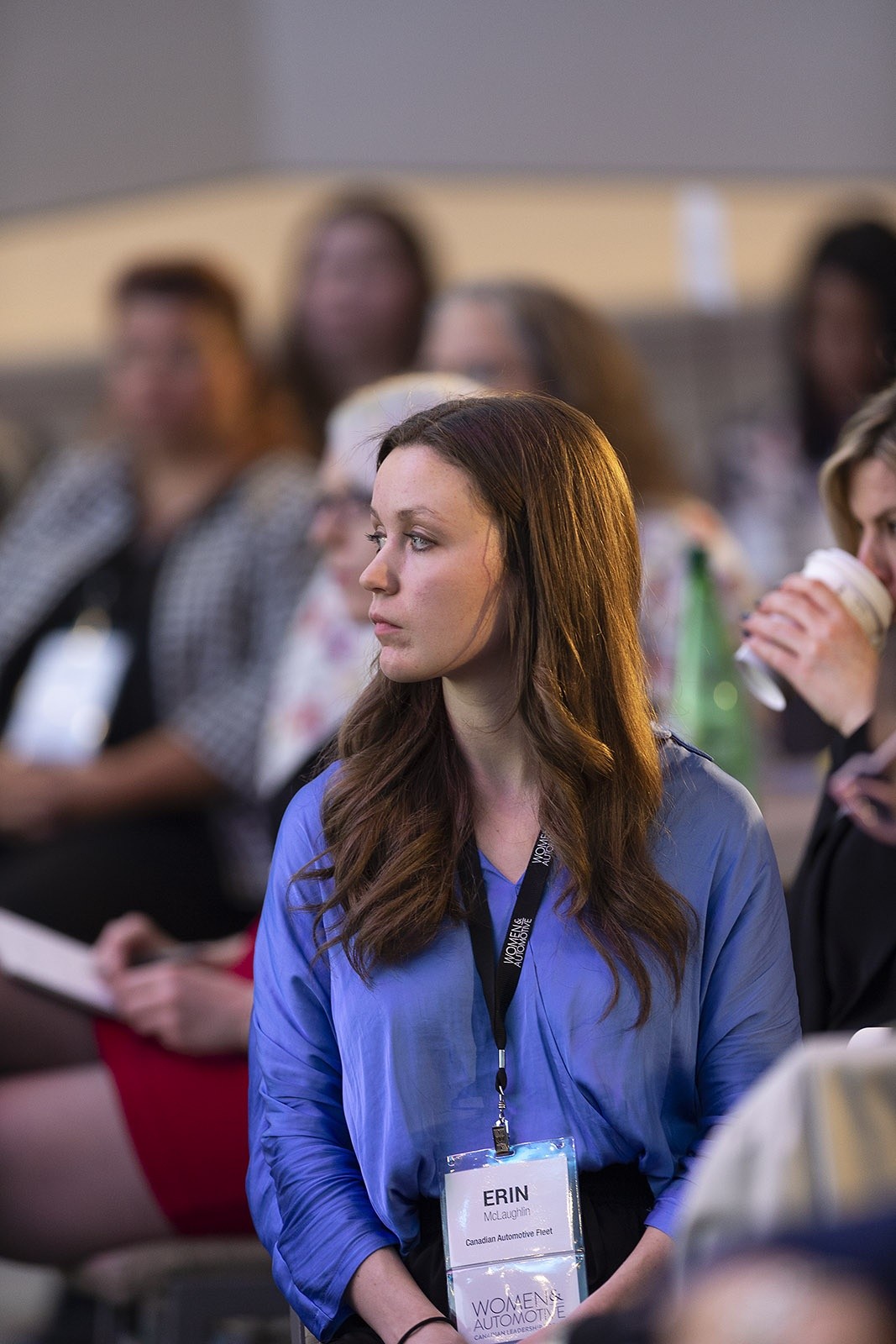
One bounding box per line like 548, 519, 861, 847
732, 546, 893, 712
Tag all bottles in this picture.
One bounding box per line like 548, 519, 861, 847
676, 542, 760, 800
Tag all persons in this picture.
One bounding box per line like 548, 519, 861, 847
0, 204, 895, 1344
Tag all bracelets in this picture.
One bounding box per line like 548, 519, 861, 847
397, 1316, 458, 1344
